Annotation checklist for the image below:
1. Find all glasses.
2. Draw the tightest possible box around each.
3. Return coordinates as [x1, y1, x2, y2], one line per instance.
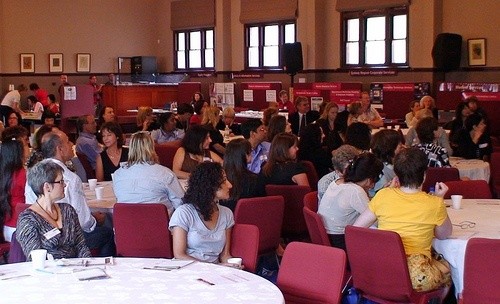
[9, 117, 18, 120]
[43, 180, 64, 187]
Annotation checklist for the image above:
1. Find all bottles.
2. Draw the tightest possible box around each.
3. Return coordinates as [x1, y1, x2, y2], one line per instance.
[346, 288, 359, 304]
[225, 125, 230, 141]
[430, 187, 434, 196]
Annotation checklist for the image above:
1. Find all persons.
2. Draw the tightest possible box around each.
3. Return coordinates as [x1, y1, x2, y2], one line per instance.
[0, 125, 116, 262]
[406, 96, 492, 167]
[137, 92, 272, 214]
[111, 130, 186, 218]
[317, 122, 452, 304]
[168, 160, 244, 268]
[0, 83, 59, 141]
[86, 73, 104, 108]
[95, 104, 128, 181]
[105, 73, 114, 85]
[59, 74, 68, 87]
[261, 90, 384, 188]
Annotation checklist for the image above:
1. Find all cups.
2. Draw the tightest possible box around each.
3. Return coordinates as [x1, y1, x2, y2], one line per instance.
[95, 187, 104, 200]
[395, 125, 399, 129]
[88, 179, 97, 191]
[451, 195, 463, 209]
[30, 249, 47, 269]
[387, 126, 391, 129]
[227, 258, 242, 267]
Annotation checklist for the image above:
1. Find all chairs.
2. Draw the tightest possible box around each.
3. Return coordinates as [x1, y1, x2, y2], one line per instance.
[0, 112, 500, 304]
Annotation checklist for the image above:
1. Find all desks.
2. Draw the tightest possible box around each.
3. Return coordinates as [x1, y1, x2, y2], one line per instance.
[21, 113, 42, 132]
[370, 128, 451, 139]
[0, 258, 285, 304]
[447, 157, 491, 184]
[369, 198, 500, 300]
[235, 110, 289, 119]
[220, 130, 245, 143]
[81, 179, 188, 214]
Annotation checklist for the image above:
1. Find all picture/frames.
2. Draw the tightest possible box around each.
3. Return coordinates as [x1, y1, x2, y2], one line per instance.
[49, 53, 64, 73]
[76, 53, 91, 73]
[467, 38, 487, 66]
[20, 53, 35, 73]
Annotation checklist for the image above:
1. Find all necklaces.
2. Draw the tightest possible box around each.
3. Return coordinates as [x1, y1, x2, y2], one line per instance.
[36, 200, 58, 221]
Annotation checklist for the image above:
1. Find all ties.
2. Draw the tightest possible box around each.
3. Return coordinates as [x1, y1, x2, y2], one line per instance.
[300, 115, 305, 137]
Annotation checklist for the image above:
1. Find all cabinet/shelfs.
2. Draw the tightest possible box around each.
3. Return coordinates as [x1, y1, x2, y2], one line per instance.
[101, 82, 201, 112]
